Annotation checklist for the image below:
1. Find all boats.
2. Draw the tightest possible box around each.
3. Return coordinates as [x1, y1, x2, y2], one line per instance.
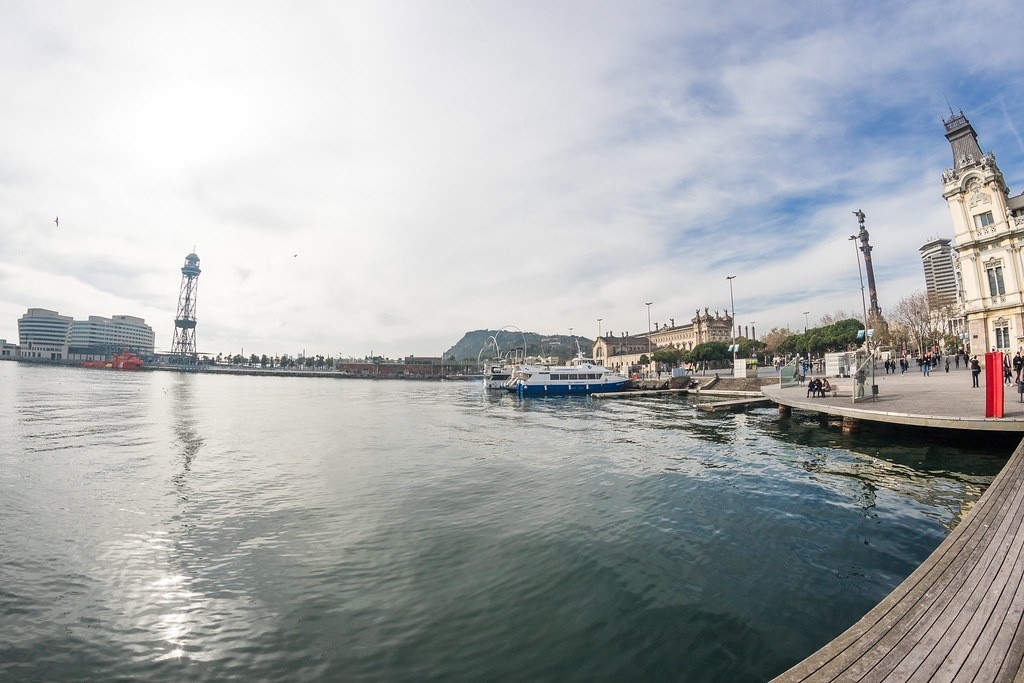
[484, 337, 631, 400]
[79, 347, 143, 369]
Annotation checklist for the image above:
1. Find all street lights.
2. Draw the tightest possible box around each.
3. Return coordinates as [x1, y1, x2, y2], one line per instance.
[803, 312, 810, 330]
[568, 327, 573, 361]
[645, 302, 653, 378]
[848, 235, 872, 358]
[727, 276, 736, 362]
[597, 319, 602, 364]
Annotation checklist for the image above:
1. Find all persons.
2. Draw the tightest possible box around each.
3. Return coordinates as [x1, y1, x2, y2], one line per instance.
[964, 351, 968, 367]
[885, 358, 895, 374]
[775, 360, 781, 371]
[1013, 347, 1024, 383]
[955, 352, 959, 368]
[916, 349, 942, 377]
[1004, 354, 1014, 387]
[799, 361, 813, 387]
[855, 370, 866, 399]
[945, 356, 951, 372]
[807, 378, 831, 398]
[900, 357, 908, 376]
[969, 355, 979, 388]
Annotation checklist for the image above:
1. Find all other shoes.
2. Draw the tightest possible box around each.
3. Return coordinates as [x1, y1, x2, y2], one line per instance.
[854, 397, 858, 399]
[1009, 383, 1014, 386]
[1014, 382, 1018, 386]
[1004, 383, 1008, 387]
[812, 396, 815, 398]
[817, 396, 819, 398]
[977, 386, 979, 387]
[859, 395, 863, 399]
[972, 386, 975, 388]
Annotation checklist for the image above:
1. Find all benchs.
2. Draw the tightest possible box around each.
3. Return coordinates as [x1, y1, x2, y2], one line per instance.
[814, 384, 839, 399]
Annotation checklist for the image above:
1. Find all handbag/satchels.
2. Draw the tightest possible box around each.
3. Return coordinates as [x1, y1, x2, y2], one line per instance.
[1012, 365, 1018, 371]
[1003, 366, 1007, 371]
[977, 365, 981, 374]
